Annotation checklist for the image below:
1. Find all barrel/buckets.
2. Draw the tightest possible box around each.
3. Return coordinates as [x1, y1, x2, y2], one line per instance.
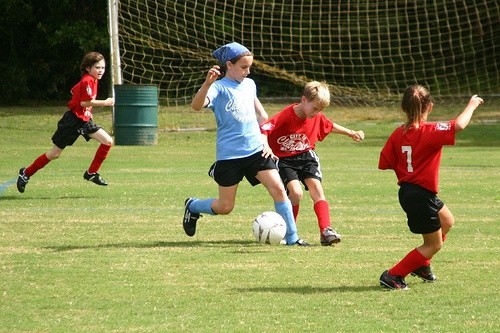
[113, 83, 159, 145]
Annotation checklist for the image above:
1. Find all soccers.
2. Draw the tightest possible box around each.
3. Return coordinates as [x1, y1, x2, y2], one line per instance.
[251, 211, 287, 245]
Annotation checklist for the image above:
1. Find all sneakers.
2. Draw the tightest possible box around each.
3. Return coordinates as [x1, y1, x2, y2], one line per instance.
[379, 271, 412, 291]
[182, 198, 203, 237]
[320, 227, 341, 246]
[287, 239, 311, 247]
[16, 167, 30, 193]
[83, 170, 108, 186]
[411, 263, 437, 283]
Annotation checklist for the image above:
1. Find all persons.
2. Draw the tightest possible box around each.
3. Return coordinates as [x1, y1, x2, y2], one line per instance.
[258, 81, 365, 245]
[17, 52, 115, 193]
[182, 43, 314, 247]
[380, 86, 484, 290]
